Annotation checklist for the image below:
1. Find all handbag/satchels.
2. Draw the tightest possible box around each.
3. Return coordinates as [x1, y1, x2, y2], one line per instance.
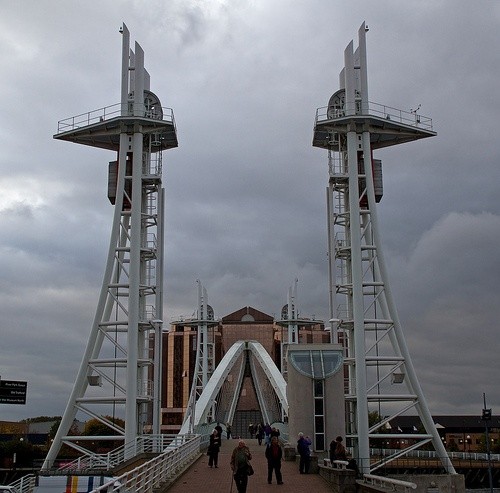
[207, 446, 212, 455]
[246, 460, 254, 476]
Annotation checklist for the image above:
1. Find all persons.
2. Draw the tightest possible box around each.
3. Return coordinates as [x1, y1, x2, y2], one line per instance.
[215, 421, 363, 479]
[208, 429, 220, 468]
[229, 440, 253, 493]
[265, 436, 284, 485]
